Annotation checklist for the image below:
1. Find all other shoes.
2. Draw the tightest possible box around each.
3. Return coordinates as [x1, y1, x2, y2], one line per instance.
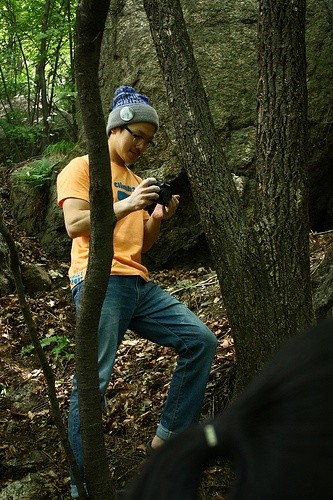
[146, 440, 169, 459]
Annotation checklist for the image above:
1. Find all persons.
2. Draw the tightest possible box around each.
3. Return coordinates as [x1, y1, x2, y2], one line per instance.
[57, 85, 218, 500]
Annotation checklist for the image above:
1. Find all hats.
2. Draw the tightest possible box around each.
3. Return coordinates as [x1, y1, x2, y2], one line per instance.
[106, 85, 159, 137]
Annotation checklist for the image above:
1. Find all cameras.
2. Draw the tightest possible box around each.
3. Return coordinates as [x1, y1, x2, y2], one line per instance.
[148, 178, 174, 206]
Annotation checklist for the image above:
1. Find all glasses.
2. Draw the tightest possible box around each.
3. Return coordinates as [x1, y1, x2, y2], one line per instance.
[124, 126, 155, 148]
